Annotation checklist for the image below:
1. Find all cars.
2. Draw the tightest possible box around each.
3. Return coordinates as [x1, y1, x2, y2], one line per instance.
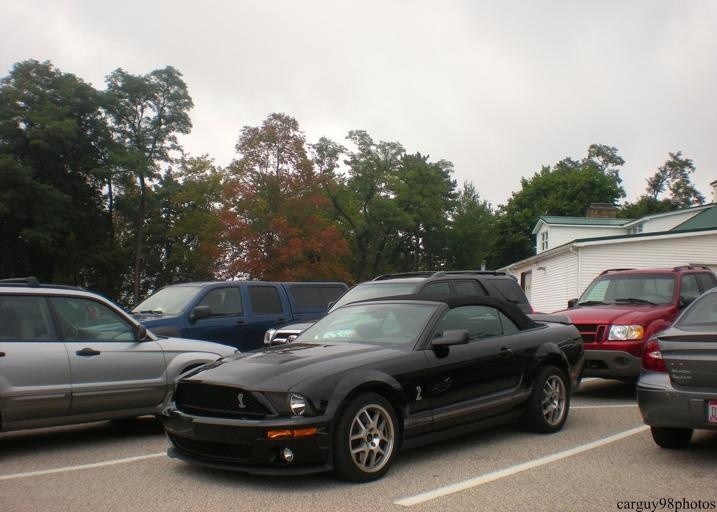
[0, 260, 715, 483]
